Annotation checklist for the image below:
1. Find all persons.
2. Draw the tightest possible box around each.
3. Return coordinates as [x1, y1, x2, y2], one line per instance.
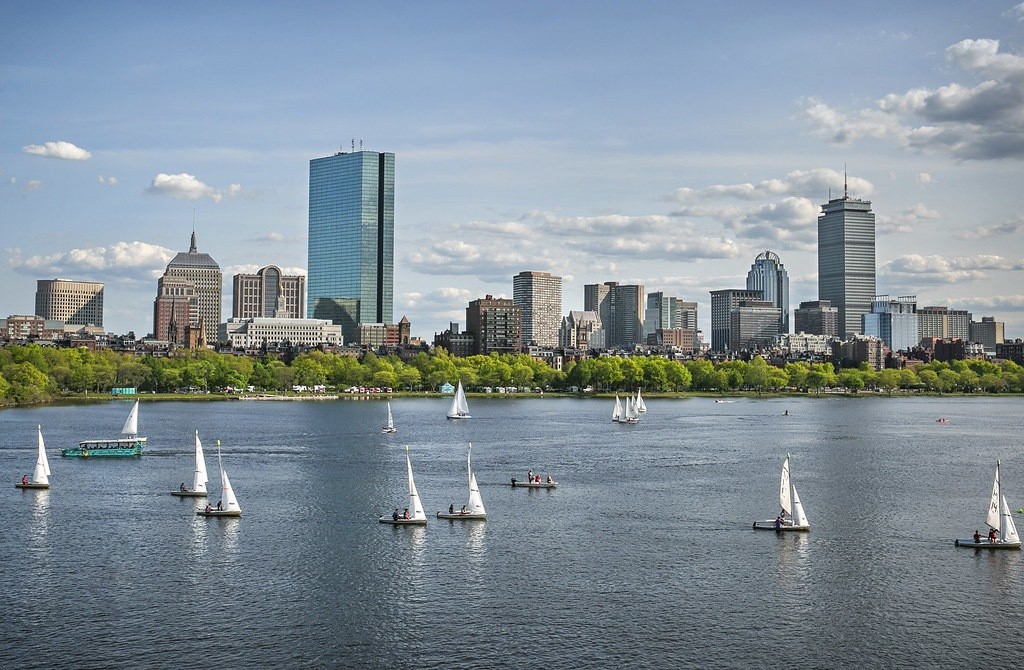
[461, 506, 469, 514]
[774, 517, 783, 526]
[780, 509, 786, 519]
[392, 509, 402, 520]
[988, 528, 998, 542]
[217, 501, 222, 511]
[404, 509, 411, 519]
[22, 475, 28, 484]
[535, 475, 541, 484]
[180, 483, 187, 493]
[205, 502, 213, 513]
[547, 476, 552, 483]
[974, 530, 981, 543]
[528, 470, 534, 482]
[449, 505, 455, 514]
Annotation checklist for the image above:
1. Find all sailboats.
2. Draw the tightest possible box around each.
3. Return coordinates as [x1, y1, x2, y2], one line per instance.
[194, 440, 242, 517]
[435, 444, 487, 519]
[171, 428, 210, 498]
[380, 400, 398, 434]
[15, 425, 54, 488]
[120, 399, 142, 446]
[377, 444, 428, 525]
[446, 379, 473, 420]
[612, 386, 646, 425]
[751, 452, 812, 530]
[954, 458, 1023, 548]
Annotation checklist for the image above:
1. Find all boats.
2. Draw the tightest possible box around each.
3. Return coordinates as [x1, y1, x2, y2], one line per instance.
[59, 436, 149, 460]
[511, 476, 559, 488]
[936, 418, 949, 423]
[713, 399, 727, 404]
